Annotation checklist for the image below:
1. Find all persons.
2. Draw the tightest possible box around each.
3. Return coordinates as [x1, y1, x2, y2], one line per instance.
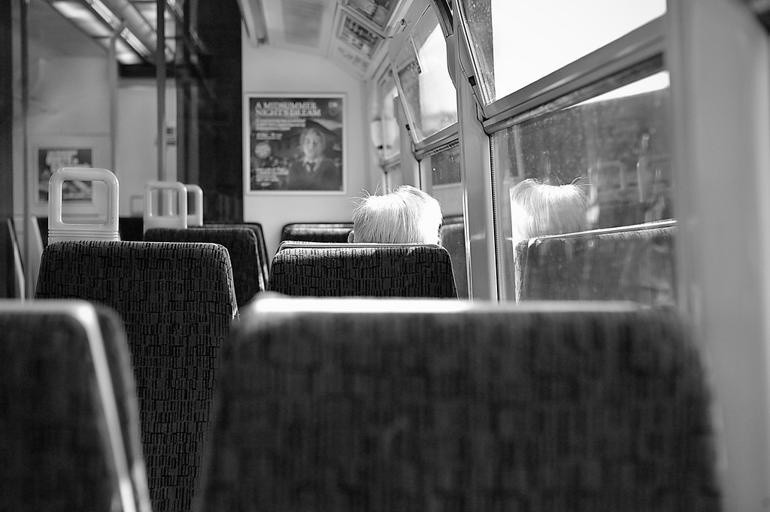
[286, 128, 341, 190]
[347, 186, 444, 245]
[509, 180, 587, 245]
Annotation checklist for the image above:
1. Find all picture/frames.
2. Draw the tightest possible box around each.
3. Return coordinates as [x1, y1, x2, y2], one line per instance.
[32, 142, 94, 206]
[244, 90, 350, 197]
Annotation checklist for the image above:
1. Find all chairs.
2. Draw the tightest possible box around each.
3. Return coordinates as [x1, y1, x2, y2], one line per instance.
[1, 207, 730, 511]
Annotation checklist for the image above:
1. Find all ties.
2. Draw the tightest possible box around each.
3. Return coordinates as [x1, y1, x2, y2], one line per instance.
[307, 161, 316, 173]
